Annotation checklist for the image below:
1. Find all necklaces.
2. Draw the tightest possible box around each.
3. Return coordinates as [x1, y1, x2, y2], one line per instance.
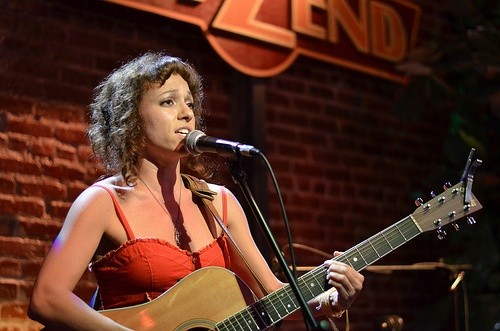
[136, 173, 190, 251]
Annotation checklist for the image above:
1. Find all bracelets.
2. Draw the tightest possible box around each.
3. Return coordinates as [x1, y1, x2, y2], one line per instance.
[315, 286, 347, 320]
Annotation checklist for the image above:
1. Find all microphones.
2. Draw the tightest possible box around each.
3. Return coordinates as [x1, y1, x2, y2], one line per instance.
[185, 130, 260, 158]
[450, 270, 466, 291]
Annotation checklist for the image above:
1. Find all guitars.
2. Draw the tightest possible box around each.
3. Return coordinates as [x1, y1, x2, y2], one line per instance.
[40, 179, 483, 331]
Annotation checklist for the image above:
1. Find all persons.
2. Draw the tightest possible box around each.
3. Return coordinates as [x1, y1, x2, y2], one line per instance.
[26, 51, 365, 331]
